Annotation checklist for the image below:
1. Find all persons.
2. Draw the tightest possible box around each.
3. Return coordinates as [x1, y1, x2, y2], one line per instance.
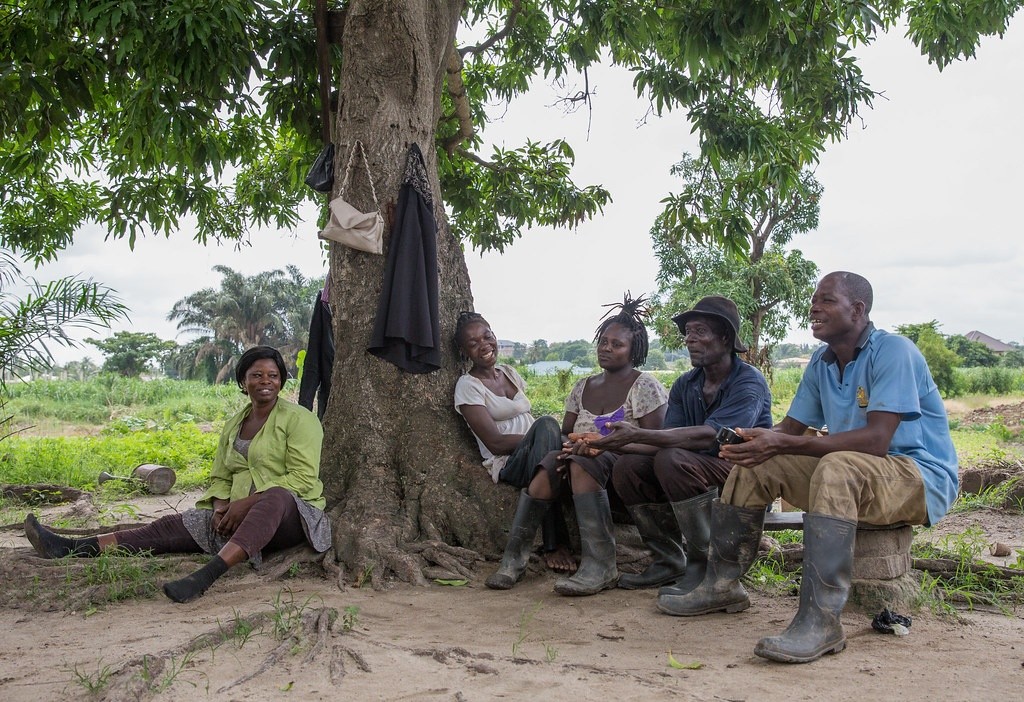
[25, 346, 331, 602]
[656, 270, 958, 661]
[571, 296, 773, 594]
[484, 289, 669, 595]
[453, 311, 577, 570]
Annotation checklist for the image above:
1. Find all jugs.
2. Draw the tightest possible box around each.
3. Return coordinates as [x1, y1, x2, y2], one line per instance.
[98, 463, 176, 494]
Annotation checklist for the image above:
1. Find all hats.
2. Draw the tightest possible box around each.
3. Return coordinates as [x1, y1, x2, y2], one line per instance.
[672, 296, 748, 355]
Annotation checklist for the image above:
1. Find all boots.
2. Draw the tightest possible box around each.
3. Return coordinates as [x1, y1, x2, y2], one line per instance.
[657, 484, 719, 598]
[656, 497, 769, 616]
[753, 512, 859, 663]
[485, 487, 556, 590]
[619, 503, 689, 590]
[555, 489, 619, 595]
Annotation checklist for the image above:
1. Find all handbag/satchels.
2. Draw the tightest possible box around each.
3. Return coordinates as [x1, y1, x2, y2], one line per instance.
[318, 139, 385, 254]
[303, 142, 336, 192]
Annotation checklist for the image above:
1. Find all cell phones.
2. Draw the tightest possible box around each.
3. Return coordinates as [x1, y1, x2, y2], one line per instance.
[716, 425, 746, 444]
